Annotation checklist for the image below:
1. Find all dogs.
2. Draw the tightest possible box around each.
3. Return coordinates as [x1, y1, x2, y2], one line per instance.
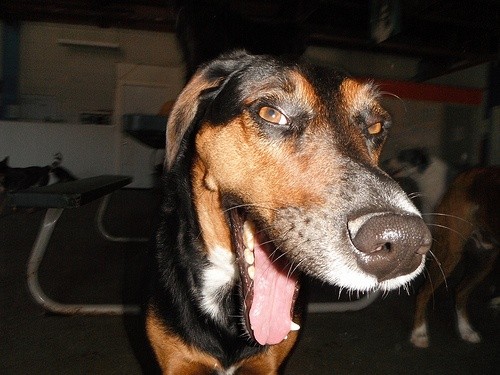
[409, 164, 499, 349]
[0, 154, 77, 214]
[144, 48, 481, 375]
[379, 144, 495, 252]
